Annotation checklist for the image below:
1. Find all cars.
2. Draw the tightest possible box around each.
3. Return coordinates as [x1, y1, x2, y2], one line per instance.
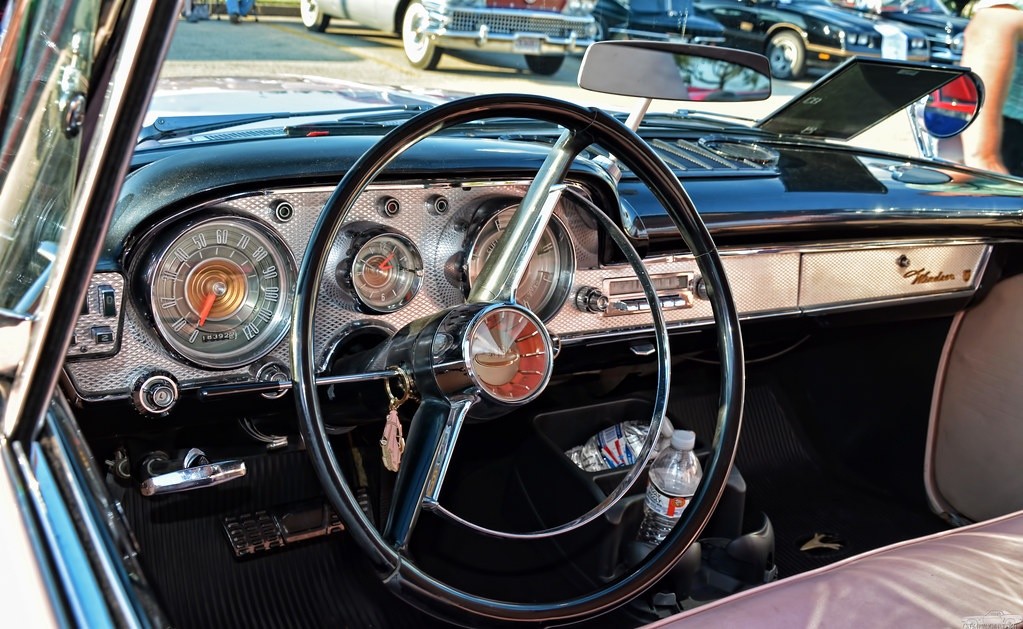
[587, 0, 971, 80]
[301, 0, 601, 76]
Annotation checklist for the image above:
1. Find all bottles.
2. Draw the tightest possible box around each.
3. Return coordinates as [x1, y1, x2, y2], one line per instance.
[636, 430, 702, 544]
[564, 415, 675, 472]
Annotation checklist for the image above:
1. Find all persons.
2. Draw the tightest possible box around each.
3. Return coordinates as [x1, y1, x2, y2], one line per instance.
[958, 0, 1023, 179]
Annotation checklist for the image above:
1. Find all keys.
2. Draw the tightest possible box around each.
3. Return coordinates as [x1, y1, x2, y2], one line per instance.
[379, 365, 411, 472]
